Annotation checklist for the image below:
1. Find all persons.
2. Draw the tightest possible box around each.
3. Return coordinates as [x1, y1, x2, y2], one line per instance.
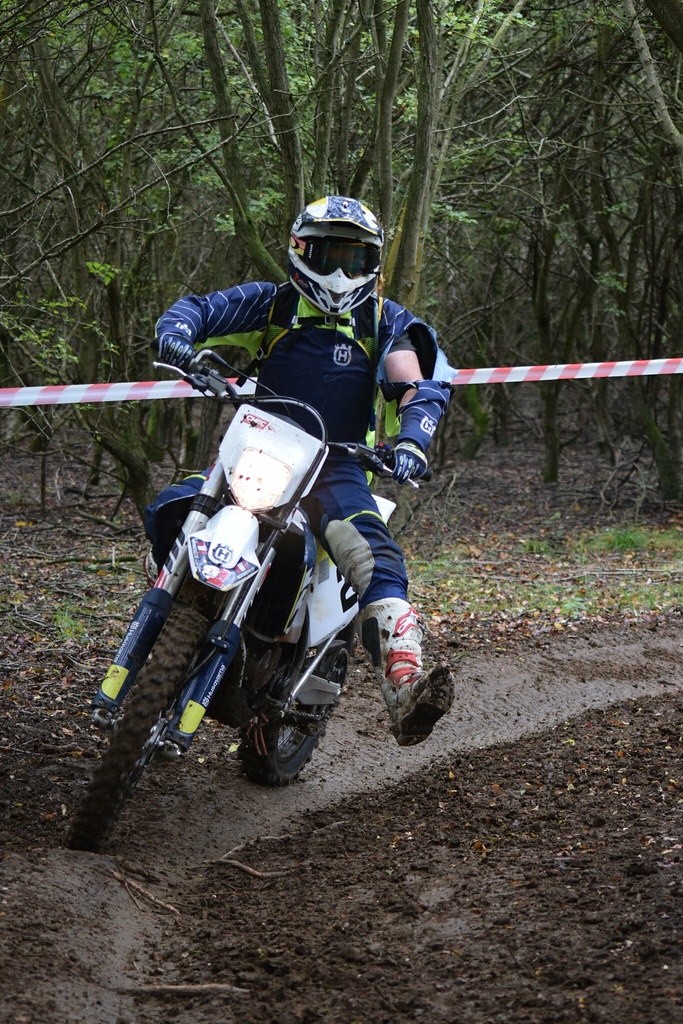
[144, 196, 455, 746]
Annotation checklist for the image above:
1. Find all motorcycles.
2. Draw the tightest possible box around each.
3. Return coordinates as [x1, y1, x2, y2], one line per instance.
[64, 338, 433, 855]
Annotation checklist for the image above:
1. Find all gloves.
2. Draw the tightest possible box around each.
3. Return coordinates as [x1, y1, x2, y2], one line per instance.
[156, 333, 195, 366]
[390, 440, 430, 484]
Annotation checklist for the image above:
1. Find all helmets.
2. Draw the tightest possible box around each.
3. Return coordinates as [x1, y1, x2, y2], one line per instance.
[287, 195, 385, 316]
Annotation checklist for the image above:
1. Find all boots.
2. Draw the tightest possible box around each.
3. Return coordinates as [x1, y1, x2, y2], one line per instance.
[363, 596, 453, 747]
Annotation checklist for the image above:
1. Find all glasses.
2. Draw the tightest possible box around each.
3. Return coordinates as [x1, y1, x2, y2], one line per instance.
[297, 238, 378, 280]
